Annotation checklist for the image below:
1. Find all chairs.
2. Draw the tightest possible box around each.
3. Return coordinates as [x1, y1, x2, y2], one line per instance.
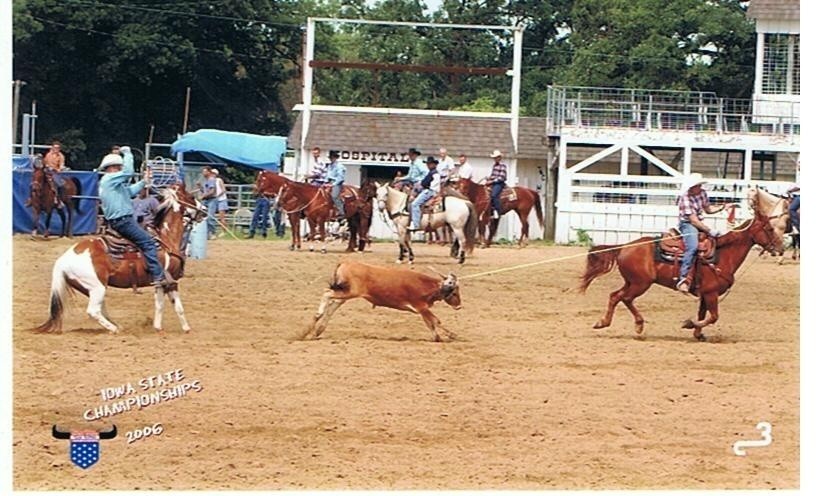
[231, 209, 252, 239]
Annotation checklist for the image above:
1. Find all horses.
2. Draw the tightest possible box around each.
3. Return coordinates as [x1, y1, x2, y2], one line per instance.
[31, 162, 83, 240]
[28, 183, 208, 338]
[251, 168, 544, 270]
[746, 183, 799, 264]
[580, 207, 786, 343]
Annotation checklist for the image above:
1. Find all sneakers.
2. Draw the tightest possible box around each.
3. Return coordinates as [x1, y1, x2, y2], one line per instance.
[155, 278, 180, 286]
[244, 233, 255, 240]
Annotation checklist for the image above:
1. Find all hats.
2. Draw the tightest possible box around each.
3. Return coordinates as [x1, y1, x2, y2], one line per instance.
[406, 148, 421, 156]
[210, 168, 220, 177]
[423, 156, 440, 165]
[98, 153, 127, 171]
[489, 149, 505, 159]
[680, 171, 707, 191]
[327, 150, 340, 159]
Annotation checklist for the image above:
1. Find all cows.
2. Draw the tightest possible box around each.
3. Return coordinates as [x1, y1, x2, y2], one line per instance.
[301, 259, 463, 344]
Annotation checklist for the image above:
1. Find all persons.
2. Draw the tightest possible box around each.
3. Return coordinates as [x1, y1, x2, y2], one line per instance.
[96, 144, 289, 240]
[675, 172, 733, 293]
[91, 146, 173, 286]
[407, 156, 442, 232]
[300, 147, 328, 186]
[448, 153, 474, 181]
[485, 149, 508, 220]
[783, 160, 800, 236]
[304, 149, 346, 220]
[393, 147, 431, 186]
[435, 147, 456, 188]
[23, 141, 66, 208]
